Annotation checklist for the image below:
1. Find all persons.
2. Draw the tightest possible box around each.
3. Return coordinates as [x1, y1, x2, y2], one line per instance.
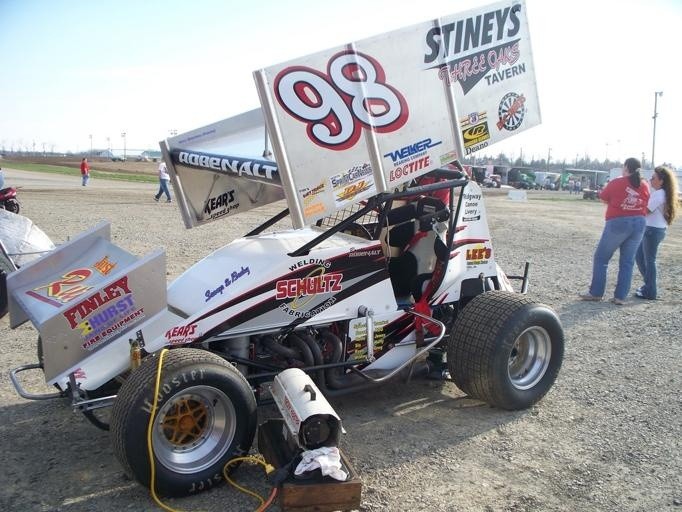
[79, 157, 90, 186]
[403, 164, 453, 207]
[153, 157, 173, 203]
[634, 165, 677, 300]
[0, 166, 4, 191]
[544, 174, 581, 195]
[578, 157, 651, 304]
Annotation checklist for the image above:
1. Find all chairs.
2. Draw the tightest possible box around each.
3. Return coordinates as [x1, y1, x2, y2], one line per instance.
[378, 197, 447, 247]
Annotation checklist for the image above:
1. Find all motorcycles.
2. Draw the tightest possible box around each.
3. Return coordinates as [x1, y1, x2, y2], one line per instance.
[1, 187, 20, 216]
[6, 2, 565, 496]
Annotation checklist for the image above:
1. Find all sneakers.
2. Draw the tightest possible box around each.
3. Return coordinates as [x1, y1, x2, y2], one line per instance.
[609, 296, 624, 304]
[636, 287, 644, 297]
[580, 294, 602, 301]
